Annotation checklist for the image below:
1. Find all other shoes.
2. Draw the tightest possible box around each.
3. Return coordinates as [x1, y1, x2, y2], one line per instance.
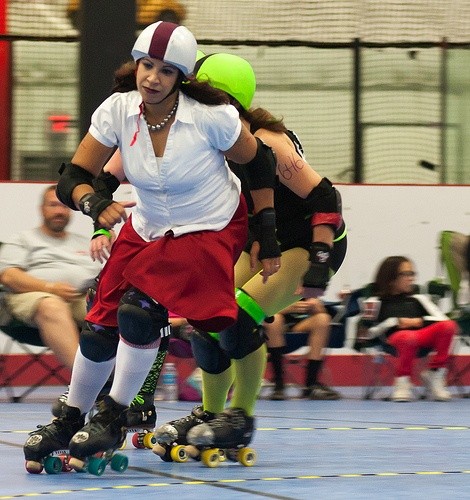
[420, 366, 454, 400]
[268, 380, 293, 400]
[388, 375, 418, 402]
[301, 383, 340, 401]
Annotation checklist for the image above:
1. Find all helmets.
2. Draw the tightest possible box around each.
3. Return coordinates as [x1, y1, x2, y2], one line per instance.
[182, 52, 256, 111]
[131, 21, 197, 80]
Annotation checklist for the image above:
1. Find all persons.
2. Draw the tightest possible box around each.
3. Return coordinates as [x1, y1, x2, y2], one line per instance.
[91, 62, 166, 449]
[24, 22, 281, 476]
[152, 52, 348, 467]
[154, 311, 202, 400]
[0, 185, 110, 372]
[358, 257, 458, 401]
[263, 300, 340, 401]
[51, 49, 207, 448]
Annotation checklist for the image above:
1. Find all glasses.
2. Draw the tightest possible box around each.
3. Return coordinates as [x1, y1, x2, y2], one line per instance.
[395, 271, 416, 277]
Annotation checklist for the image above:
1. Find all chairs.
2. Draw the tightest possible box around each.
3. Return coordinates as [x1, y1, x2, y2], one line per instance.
[0, 278, 470, 403]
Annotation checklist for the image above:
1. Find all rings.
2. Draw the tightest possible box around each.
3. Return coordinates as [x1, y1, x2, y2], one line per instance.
[276, 264, 280, 266]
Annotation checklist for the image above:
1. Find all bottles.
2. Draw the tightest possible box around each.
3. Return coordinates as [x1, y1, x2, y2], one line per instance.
[160, 362, 179, 400]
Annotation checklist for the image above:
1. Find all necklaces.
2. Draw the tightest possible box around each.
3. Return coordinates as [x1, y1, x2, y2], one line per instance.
[143, 97, 178, 130]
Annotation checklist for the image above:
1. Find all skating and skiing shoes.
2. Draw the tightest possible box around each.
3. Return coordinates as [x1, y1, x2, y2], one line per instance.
[62, 396, 134, 477]
[151, 407, 222, 464]
[170, 406, 258, 468]
[51, 383, 74, 420]
[118, 393, 159, 449]
[21, 402, 87, 475]
